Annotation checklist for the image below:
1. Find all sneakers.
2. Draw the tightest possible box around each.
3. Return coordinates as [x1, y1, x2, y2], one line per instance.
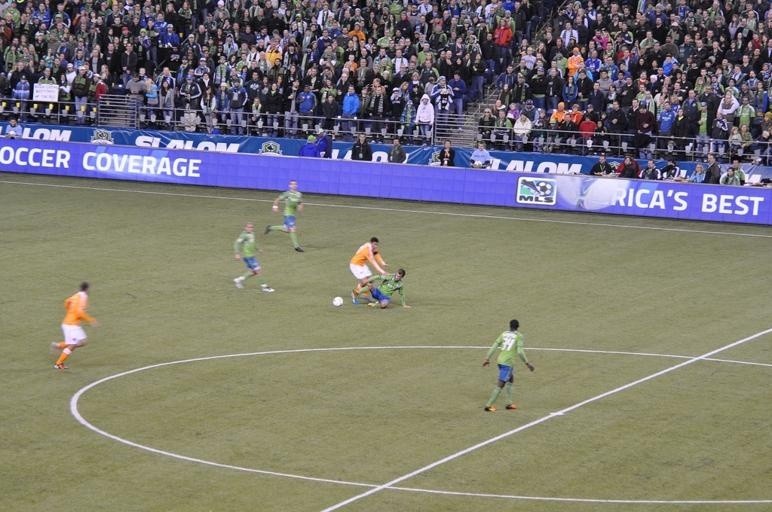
[264, 225, 271, 235]
[50, 341, 59, 353]
[233, 278, 245, 289]
[295, 247, 304, 252]
[505, 403, 519, 411]
[261, 283, 275, 293]
[54, 363, 69, 371]
[351, 288, 380, 307]
[485, 404, 498, 412]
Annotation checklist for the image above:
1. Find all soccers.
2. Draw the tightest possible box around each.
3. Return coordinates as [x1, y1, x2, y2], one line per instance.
[332, 296, 345, 307]
[536, 182, 553, 197]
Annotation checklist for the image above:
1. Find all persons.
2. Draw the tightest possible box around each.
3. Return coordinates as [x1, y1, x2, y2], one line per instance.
[481, 318, 536, 412]
[701, 154, 721, 185]
[719, 167, 742, 186]
[591, 153, 612, 176]
[637, 159, 661, 179]
[351, 268, 411, 309]
[0, 0, 771, 161]
[612, 153, 640, 174]
[439, 140, 455, 167]
[349, 235, 389, 305]
[469, 142, 491, 170]
[688, 165, 706, 184]
[733, 159, 747, 186]
[658, 160, 679, 180]
[619, 154, 639, 177]
[49, 283, 99, 374]
[264, 179, 305, 254]
[232, 221, 275, 293]
